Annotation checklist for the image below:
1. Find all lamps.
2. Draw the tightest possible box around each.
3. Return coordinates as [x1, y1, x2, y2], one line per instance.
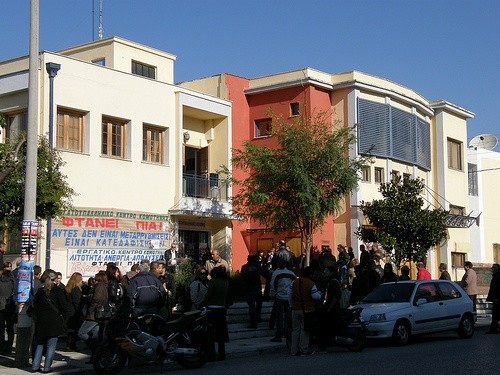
[183, 131, 189, 140]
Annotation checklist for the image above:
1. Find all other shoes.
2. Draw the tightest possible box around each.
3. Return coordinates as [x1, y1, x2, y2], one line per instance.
[485, 330, 497, 334]
[271, 337, 282, 342]
[32, 369, 41, 372]
[44, 370, 53, 372]
[248, 324, 256, 328]
[301, 351, 314, 356]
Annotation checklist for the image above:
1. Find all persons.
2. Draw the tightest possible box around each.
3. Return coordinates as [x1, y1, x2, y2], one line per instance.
[65, 272, 84, 351]
[201, 266, 230, 363]
[438, 263, 452, 281]
[322, 278, 343, 314]
[26, 273, 68, 373]
[241, 253, 269, 329]
[416, 262, 431, 281]
[77, 273, 108, 365]
[457, 262, 477, 324]
[381, 263, 398, 283]
[0, 241, 7, 269]
[0, 262, 17, 356]
[398, 266, 412, 281]
[80, 242, 231, 329]
[269, 257, 298, 343]
[289, 266, 321, 357]
[255, 240, 381, 300]
[28, 266, 67, 357]
[485, 264, 500, 334]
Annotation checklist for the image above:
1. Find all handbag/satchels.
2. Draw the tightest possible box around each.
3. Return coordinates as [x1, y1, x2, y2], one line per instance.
[55, 313, 67, 334]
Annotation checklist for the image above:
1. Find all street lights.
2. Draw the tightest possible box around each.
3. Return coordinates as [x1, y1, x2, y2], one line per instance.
[45, 62, 62, 268]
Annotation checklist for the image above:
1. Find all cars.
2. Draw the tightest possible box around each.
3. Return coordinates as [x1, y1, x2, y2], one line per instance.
[345, 279, 478, 346]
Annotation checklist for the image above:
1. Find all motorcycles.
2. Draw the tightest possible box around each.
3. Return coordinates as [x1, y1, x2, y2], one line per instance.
[91, 301, 214, 375]
[285, 307, 369, 353]
